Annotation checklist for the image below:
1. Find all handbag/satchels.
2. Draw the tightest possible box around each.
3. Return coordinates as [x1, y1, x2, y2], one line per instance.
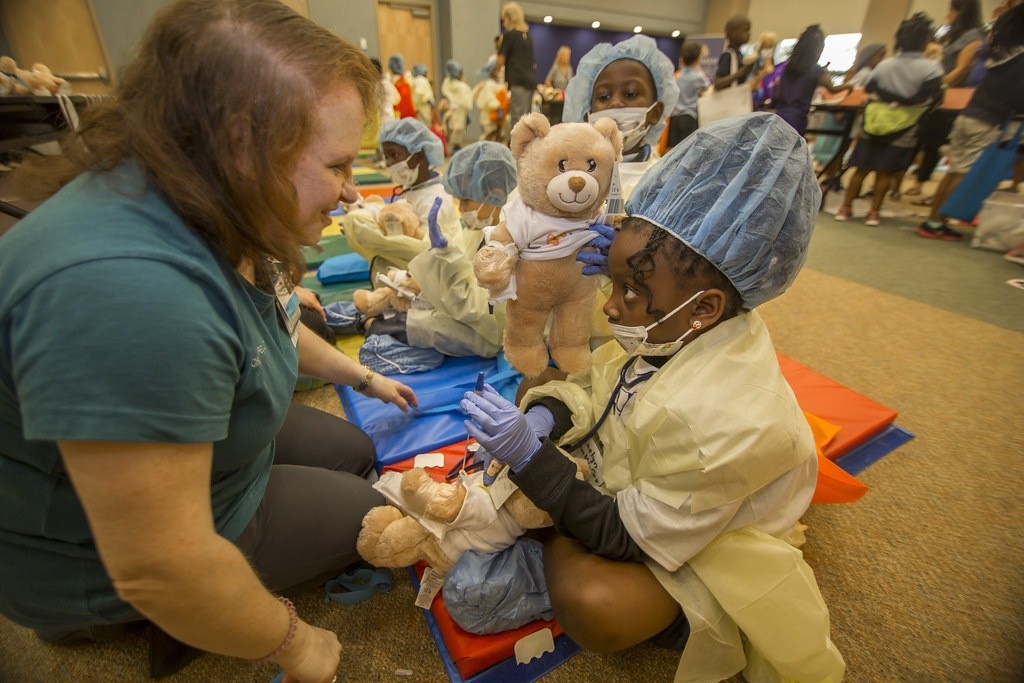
[863, 98, 935, 144]
[698, 45, 753, 127]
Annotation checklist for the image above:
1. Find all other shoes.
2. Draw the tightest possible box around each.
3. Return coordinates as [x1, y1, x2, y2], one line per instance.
[834, 208, 852, 221]
[865, 210, 881, 226]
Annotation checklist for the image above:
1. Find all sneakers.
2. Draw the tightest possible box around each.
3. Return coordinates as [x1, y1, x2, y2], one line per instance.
[916, 221, 964, 241]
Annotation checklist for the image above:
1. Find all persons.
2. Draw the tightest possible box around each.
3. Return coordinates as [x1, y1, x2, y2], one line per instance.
[716, 1, 1024, 241]
[344, 35, 846, 683]
[0, 1, 419, 683]
[491, 3, 536, 128]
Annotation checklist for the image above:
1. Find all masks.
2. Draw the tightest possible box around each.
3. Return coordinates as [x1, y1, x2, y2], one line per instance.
[606, 290, 712, 357]
[460, 193, 497, 231]
[383, 150, 422, 190]
[588, 102, 660, 152]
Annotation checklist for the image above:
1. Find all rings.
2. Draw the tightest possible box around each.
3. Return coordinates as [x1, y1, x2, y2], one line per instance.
[332, 675, 337, 683]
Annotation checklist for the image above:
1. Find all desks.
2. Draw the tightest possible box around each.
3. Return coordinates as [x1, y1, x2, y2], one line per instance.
[758, 87, 975, 213]
[0, 91, 114, 220]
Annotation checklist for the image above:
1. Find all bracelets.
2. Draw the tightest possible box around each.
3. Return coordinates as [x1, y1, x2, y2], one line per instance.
[254, 596, 298, 664]
[352, 370, 373, 393]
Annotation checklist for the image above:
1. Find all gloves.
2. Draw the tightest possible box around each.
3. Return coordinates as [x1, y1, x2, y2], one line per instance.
[460, 382, 543, 472]
[474, 407, 555, 486]
[497, 107, 502, 118]
[427, 196, 448, 248]
[466, 114, 472, 125]
[429, 100, 434, 108]
[576, 224, 616, 276]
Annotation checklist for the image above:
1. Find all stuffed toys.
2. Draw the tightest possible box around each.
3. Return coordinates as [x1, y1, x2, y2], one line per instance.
[358, 447, 590, 575]
[339, 194, 423, 241]
[0, 56, 61, 87]
[476, 113, 623, 376]
[354, 265, 421, 317]
